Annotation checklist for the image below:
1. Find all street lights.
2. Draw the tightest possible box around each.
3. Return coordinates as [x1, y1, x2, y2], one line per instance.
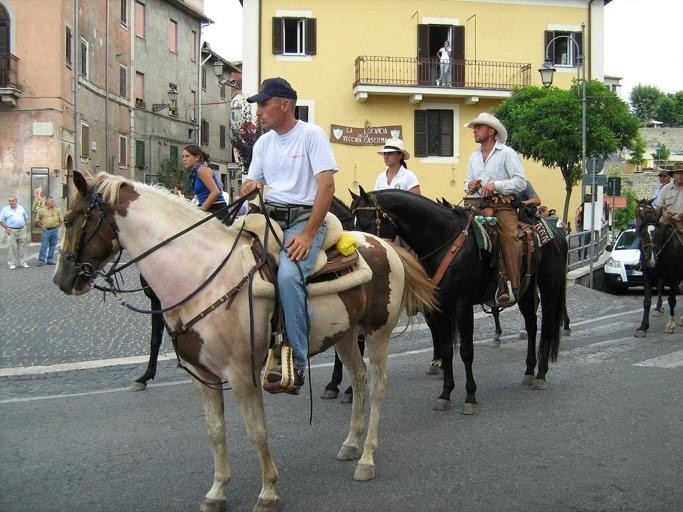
[537, 32, 588, 261]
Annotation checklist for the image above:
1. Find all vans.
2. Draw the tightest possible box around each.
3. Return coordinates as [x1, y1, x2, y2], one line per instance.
[601, 225, 656, 296]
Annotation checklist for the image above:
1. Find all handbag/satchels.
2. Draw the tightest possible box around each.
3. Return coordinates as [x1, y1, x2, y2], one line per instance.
[263, 202, 313, 229]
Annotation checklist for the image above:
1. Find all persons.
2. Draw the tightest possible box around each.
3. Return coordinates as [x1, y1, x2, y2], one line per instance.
[33, 196, 63, 265]
[217, 181, 228, 205]
[0, 196, 29, 269]
[632, 161, 682, 273]
[461, 112, 527, 306]
[171, 184, 181, 195]
[228, 195, 244, 217]
[575, 203, 589, 262]
[435, 41, 452, 87]
[182, 145, 228, 223]
[372, 139, 419, 194]
[652, 170, 671, 208]
[547, 208, 556, 218]
[237, 77, 338, 394]
[539, 206, 545, 218]
[31, 185, 47, 227]
[515, 179, 541, 208]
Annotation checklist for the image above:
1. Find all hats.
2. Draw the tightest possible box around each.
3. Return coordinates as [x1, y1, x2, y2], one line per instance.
[668, 163, 683, 178]
[657, 171, 668, 176]
[464, 112, 508, 144]
[247, 77, 297, 103]
[376, 138, 410, 160]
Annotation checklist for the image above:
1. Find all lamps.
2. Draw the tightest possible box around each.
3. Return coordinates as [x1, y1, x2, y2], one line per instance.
[153, 87, 178, 112]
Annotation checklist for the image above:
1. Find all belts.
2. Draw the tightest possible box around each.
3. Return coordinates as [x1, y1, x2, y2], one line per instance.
[10, 227, 23, 229]
[46, 227, 57, 230]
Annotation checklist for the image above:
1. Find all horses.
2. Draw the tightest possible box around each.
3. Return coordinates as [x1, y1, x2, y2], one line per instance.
[348, 186, 568, 415]
[634, 209, 683, 338]
[53, 171, 444, 512]
[631, 196, 659, 235]
[319, 180, 444, 404]
[435, 196, 571, 348]
[125, 272, 164, 393]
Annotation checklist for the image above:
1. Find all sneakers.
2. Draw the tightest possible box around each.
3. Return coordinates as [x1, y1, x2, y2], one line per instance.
[499, 288, 519, 302]
[268, 366, 304, 395]
[9, 261, 56, 270]
